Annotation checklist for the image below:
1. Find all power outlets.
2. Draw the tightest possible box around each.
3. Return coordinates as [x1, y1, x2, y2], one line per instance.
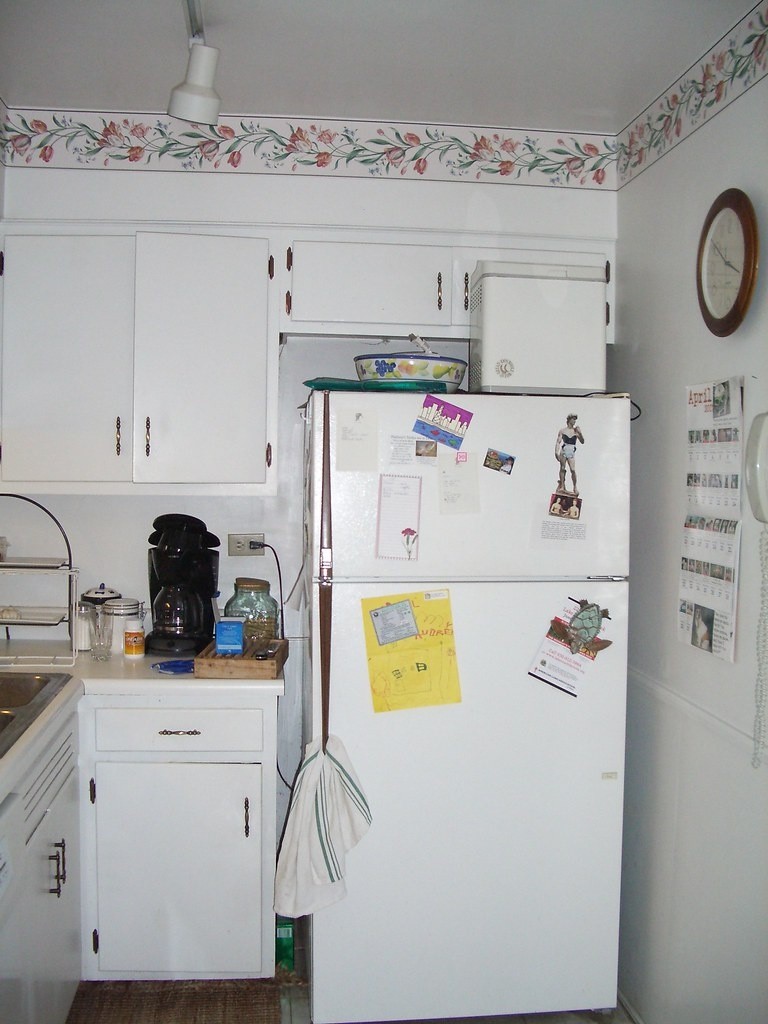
[228, 533, 265, 557]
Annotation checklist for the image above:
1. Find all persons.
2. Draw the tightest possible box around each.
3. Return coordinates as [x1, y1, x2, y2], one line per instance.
[551, 498, 579, 518]
[500, 456, 515, 475]
[554, 413, 585, 495]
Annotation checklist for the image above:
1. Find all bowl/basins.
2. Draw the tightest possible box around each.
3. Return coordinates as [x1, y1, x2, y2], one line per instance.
[354, 352, 468, 394]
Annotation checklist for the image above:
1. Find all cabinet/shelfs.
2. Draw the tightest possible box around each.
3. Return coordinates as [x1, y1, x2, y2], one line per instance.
[281, 225, 617, 346]
[0, 221, 276, 498]
[0, 694, 278, 1024]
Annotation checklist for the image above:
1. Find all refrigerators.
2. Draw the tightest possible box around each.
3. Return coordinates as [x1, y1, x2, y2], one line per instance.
[300, 389, 631, 1024]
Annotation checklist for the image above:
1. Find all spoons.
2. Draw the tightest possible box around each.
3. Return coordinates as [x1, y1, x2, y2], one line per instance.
[244, 634, 280, 660]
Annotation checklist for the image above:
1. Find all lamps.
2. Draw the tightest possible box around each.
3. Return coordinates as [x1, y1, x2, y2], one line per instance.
[166, 0, 222, 127]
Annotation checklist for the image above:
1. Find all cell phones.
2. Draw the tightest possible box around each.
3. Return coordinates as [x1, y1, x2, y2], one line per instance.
[256, 649, 267, 660]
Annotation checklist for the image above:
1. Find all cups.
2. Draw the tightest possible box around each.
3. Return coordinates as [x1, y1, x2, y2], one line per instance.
[89, 609, 113, 662]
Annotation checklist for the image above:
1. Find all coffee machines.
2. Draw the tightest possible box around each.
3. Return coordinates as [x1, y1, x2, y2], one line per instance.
[143, 513, 220, 656]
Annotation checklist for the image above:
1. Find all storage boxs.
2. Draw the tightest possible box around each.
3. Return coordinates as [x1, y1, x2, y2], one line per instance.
[470, 259, 607, 399]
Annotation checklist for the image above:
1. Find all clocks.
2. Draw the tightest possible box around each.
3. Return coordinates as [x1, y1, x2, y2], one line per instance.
[695, 188, 759, 338]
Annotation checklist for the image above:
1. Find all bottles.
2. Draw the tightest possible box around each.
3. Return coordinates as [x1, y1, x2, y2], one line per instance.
[81, 583, 146, 660]
[71, 601, 96, 650]
[224, 577, 278, 639]
